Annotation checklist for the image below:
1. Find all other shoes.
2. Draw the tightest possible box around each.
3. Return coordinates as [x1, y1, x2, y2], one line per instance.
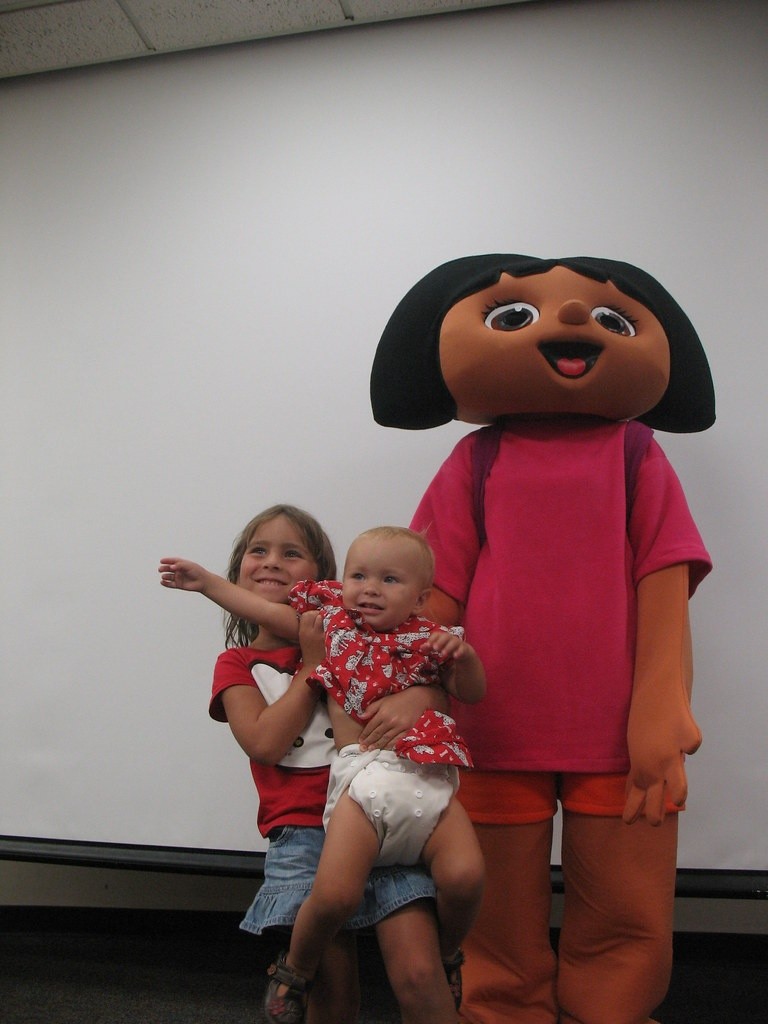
[265, 951, 319, 1024]
[441, 947, 464, 1011]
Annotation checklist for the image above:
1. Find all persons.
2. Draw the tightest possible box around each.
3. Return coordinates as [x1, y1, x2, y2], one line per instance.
[158, 525, 484, 1024]
[208, 504, 458, 1024]
[369, 253, 716, 1024]
